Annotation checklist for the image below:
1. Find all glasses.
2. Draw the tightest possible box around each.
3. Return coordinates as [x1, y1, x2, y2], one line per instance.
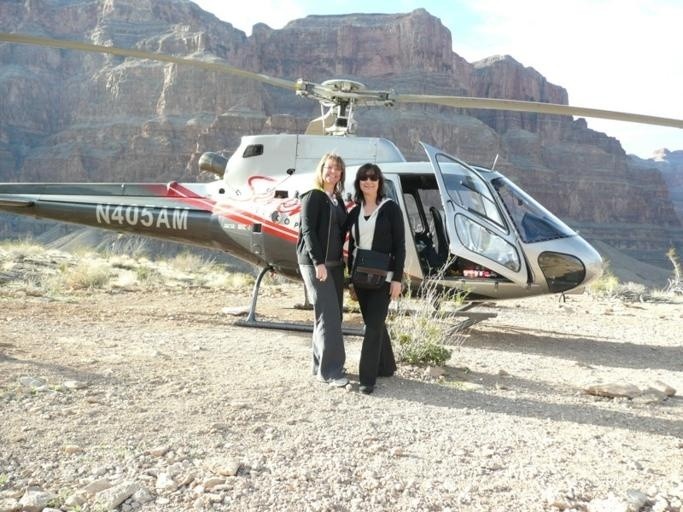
[360, 174, 379, 181]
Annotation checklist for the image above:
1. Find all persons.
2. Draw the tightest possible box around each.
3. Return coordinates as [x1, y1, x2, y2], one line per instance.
[293, 152, 351, 387]
[346, 163, 407, 394]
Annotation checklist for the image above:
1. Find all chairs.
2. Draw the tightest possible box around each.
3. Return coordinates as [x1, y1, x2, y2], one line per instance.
[430, 207, 476, 276]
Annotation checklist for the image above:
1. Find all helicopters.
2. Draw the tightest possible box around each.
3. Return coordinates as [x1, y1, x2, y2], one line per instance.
[0, 33, 683, 341]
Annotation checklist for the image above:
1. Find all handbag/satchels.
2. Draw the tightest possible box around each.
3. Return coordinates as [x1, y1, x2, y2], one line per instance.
[352, 249, 390, 290]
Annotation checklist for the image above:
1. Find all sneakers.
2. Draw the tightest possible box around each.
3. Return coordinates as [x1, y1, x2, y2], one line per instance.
[377, 371, 394, 377]
[316, 373, 348, 386]
[313, 363, 346, 376]
[360, 384, 374, 394]
